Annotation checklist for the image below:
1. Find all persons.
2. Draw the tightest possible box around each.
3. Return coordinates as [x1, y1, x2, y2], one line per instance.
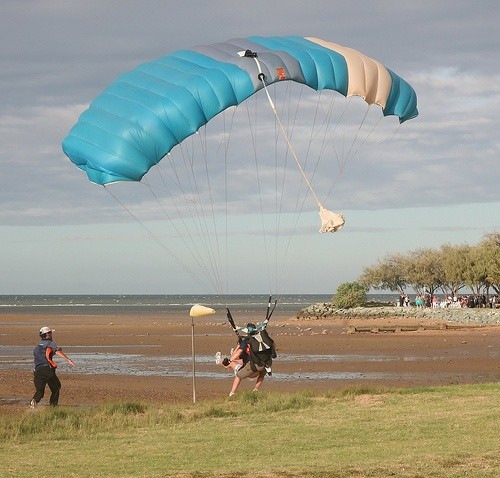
[228, 323, 276, 396]
[30, 326, 77, 410]
[215, 343, 260, 379]
[398, 291, 500, 310]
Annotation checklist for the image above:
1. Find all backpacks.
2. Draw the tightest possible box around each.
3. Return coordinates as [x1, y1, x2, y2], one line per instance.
[251, 331, 273, 363]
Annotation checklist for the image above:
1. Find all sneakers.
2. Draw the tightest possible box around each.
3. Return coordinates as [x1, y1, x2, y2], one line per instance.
[29, 399, 37, 412]
[230, 348, 235, 355]
[252, 388, 259, 391]
[215, 352, 221, 364]
[228, 392, 234, 397]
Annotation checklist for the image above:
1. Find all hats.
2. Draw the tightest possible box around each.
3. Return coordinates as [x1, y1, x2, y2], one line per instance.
[39, 326, 55, 335]
